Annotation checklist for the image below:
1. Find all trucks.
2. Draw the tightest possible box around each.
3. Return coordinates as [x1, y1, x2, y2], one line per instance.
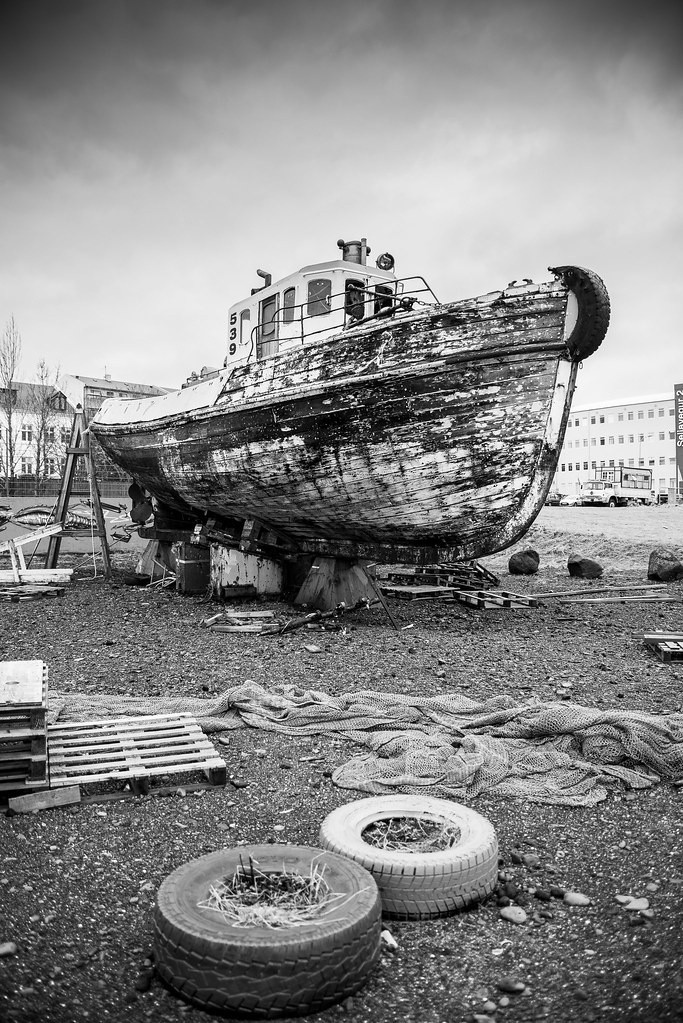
[578, 466, 653, 508]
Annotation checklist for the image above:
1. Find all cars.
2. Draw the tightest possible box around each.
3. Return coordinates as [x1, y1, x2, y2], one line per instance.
[655, 494, 668, 505]
[560, 494, 582, 507]
[546, 493, 559, 506]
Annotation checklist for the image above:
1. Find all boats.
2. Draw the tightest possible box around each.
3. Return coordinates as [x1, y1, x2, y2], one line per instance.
[87, 235, 611, 563]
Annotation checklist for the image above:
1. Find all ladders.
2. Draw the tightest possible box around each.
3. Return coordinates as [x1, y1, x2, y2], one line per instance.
[0, 521, 75, 584]
[45, 406, 114, 581]
